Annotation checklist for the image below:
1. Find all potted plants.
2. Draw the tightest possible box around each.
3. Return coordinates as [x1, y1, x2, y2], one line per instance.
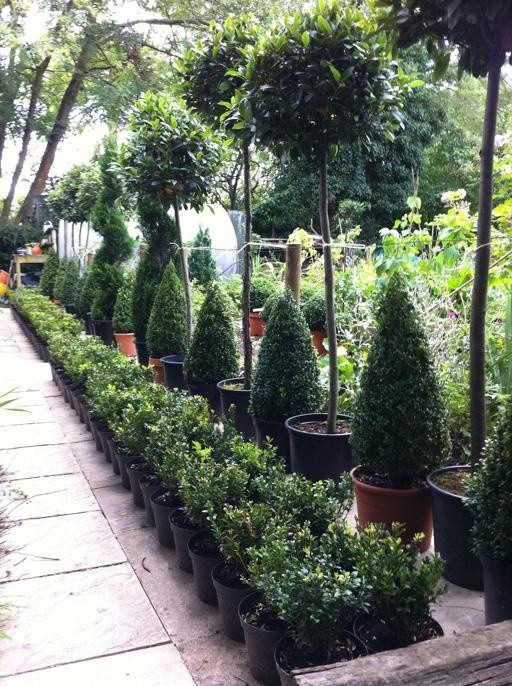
[377, 0, 511, 603]
[52, 93, 234, 401]
[223, 2, 408, 500]
[10, 282, 86, 368]
[345, 260, 457, 554]
[170, 6, 282, 447]
[463, 393, 512, 628]
[50, 326, 445, 686]
